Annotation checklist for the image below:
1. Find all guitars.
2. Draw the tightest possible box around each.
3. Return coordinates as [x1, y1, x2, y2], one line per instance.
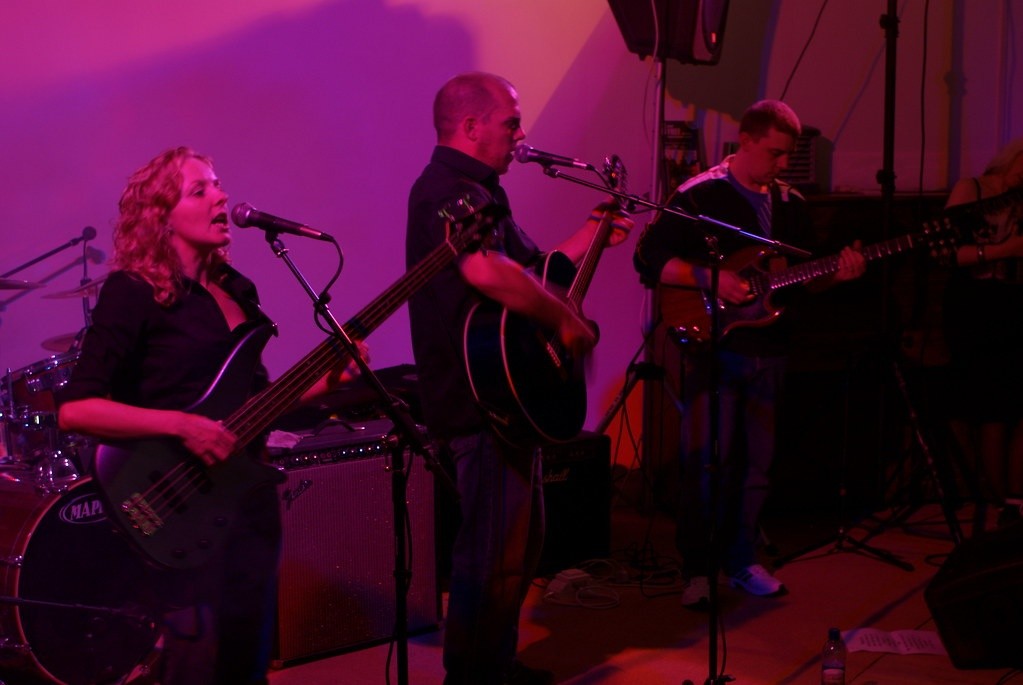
[91, 191, 504, 578]
[461, 152, 629, 451]
[658, 215, 964, 350]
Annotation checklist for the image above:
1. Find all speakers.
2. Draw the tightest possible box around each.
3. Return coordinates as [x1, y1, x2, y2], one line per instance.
[923, 521, 1023, 669]
[607, 0, 667, 57]
[262, 418, 442, 671]
[531, 430, 610, 578]
[667, 0, 731, 66]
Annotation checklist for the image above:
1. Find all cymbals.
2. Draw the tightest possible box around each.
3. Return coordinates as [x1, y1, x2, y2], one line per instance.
[41, 330, 86, 352]
[40, 286, 103, 299]
[0, 277, 44, 289]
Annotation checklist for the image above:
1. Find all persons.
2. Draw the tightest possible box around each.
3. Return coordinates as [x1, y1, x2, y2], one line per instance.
[59, 146, 369, 685]
[630, 99, 871, 610]
[404, 69, 634, 685]
[946, 137, 1023, 506]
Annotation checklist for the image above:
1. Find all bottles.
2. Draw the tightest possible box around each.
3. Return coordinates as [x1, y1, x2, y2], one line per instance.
[820, 626, 847, 685]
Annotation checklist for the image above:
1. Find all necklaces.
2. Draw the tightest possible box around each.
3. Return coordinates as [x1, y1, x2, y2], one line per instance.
[977, 243, 984, 260]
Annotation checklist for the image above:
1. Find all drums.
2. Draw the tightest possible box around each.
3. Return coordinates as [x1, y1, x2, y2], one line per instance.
[2, 349, 98, 455]
[1, 467, 170, 685]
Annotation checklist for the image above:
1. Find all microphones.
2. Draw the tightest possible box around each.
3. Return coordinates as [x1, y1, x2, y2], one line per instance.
[513, 143, 594, 171]
[231, 201, 332, 242]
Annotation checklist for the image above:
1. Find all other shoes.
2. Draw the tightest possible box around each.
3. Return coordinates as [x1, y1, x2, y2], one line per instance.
[507, 661, 552, 685]
[735, 565, 786, 597]
[681, 577, 711, 610]
